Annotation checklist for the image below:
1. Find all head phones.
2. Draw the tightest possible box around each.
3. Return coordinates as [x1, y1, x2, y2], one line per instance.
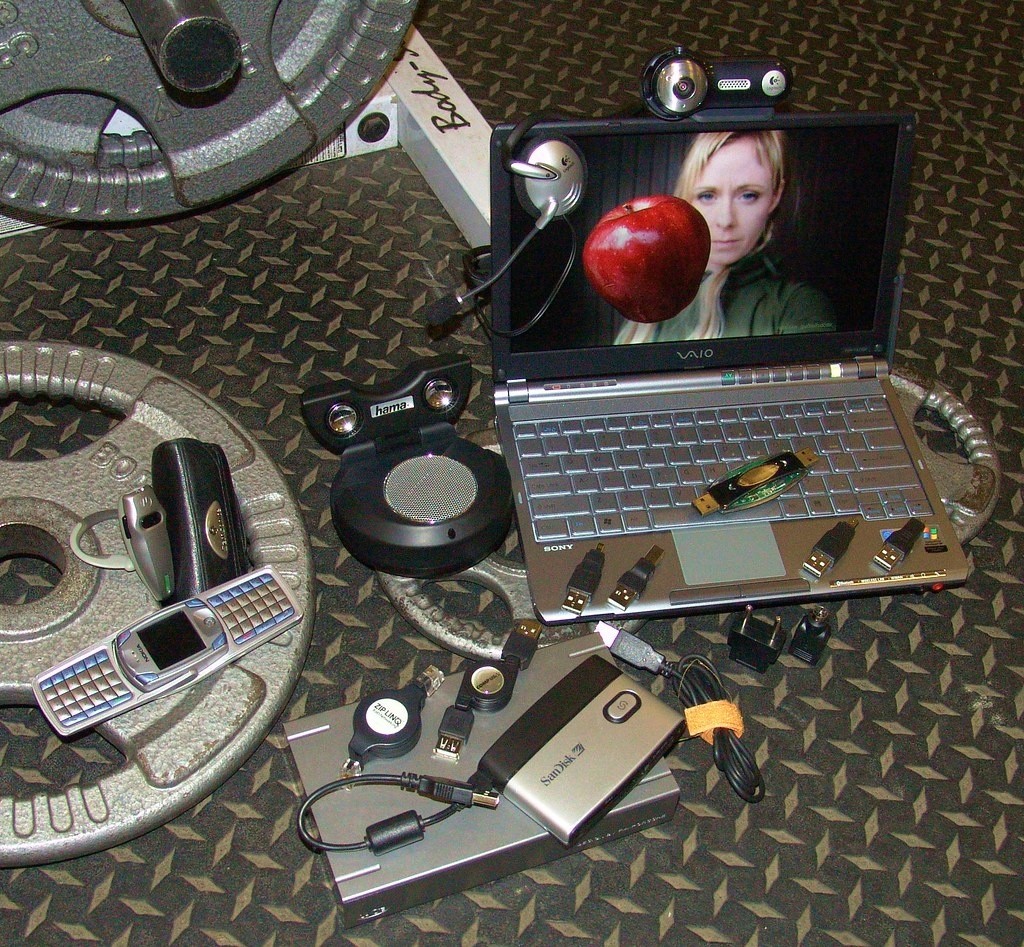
[500, 111, 589, 229]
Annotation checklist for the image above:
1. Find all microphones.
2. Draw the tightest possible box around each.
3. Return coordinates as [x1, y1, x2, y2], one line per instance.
[426, 227, 542, 327]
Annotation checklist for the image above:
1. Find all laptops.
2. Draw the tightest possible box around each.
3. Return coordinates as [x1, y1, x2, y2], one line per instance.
[487, 103, 968, 628]
